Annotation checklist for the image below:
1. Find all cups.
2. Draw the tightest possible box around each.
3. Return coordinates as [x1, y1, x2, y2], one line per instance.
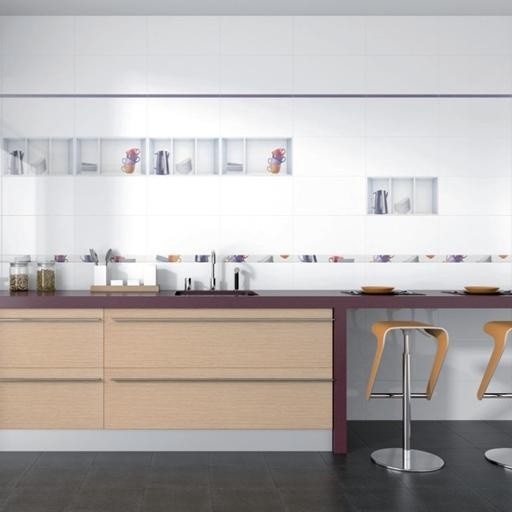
[266, 148, 287, 174]
[371, 189, 389, 214]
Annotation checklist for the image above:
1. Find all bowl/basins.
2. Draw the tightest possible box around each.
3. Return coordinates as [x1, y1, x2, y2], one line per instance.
[394, 198, 410, 214]
[176, 159, 192, 174]
[31, 159, 46, 174]
[120, 148, 141, 173]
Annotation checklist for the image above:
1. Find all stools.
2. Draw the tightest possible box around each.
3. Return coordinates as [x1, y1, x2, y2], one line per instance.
[365, 318, 512, 473]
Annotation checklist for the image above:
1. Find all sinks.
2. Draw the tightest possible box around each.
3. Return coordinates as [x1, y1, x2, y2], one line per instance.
[174, 290, 258, 295]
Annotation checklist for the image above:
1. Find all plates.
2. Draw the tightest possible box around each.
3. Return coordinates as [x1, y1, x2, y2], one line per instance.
[464, 285, 499, 292]
[81, 163, 96, 175]
[227, 161, 244, 174]
[361, 286, 394, 292]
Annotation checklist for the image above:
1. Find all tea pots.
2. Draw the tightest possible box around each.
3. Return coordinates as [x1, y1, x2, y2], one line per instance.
[153, 149, 171, 174]
[7, 150, 25, 175]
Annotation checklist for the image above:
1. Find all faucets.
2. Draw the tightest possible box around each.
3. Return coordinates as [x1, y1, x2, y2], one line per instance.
[209, 251, 216, 293]
